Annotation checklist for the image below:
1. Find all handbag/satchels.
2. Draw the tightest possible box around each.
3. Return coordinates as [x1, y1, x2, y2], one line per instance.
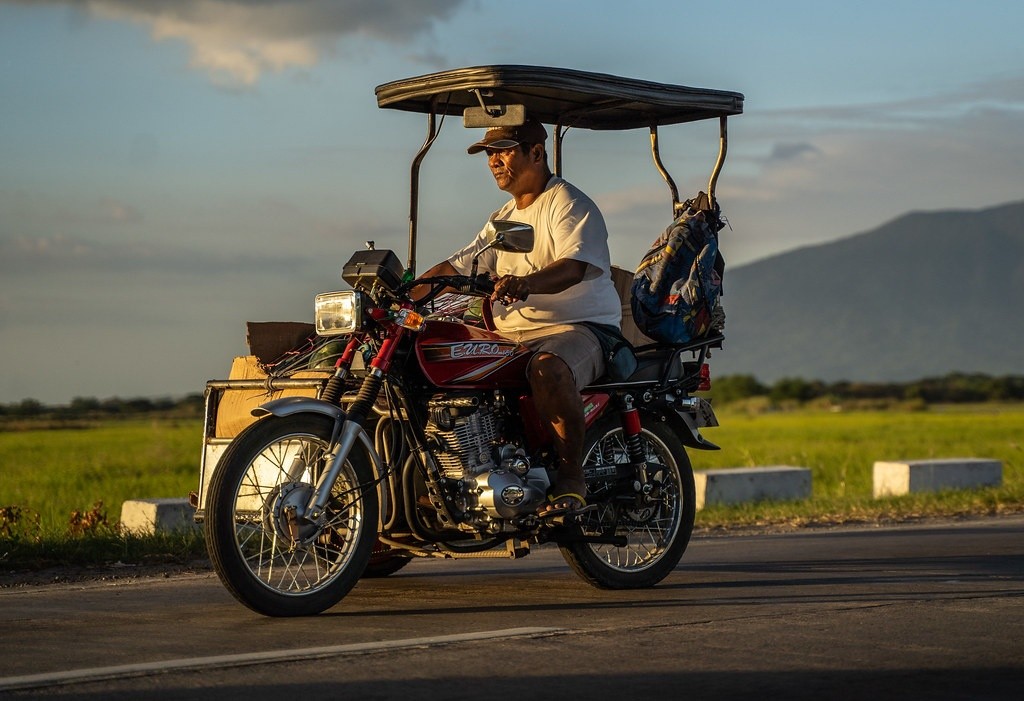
[629, 200, 725, 347]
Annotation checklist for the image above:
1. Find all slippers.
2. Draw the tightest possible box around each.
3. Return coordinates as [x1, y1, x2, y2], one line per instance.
[538, 493, 599, 517]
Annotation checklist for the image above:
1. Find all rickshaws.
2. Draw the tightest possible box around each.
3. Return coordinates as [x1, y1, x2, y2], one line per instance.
[189, 61, 746, 619]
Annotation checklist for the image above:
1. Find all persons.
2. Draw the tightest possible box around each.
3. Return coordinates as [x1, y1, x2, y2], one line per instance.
[407, 117, 627, 516]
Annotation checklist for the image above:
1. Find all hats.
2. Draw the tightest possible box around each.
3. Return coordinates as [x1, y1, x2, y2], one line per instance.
[467, 119, 548, 155]
